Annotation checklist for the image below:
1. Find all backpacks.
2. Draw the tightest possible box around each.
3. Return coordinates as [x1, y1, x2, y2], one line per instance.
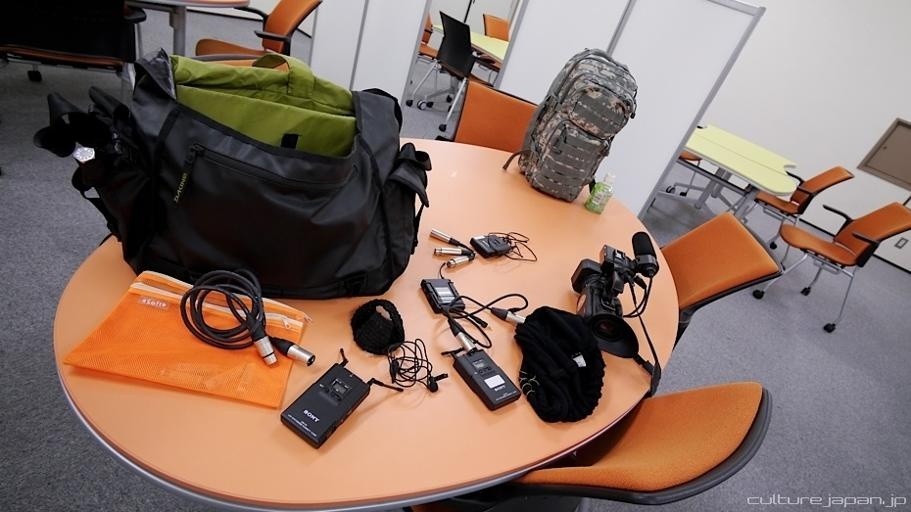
[517, 44, 641, 206]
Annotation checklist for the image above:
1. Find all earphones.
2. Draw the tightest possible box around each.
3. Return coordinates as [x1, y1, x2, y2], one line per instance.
[392, 360, 399, 378]
[427, 374, 438, 392]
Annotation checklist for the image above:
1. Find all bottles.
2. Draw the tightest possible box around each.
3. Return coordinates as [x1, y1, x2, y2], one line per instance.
[584, 169, 617, 215]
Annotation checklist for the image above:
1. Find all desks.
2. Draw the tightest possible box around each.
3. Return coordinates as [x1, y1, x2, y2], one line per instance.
[54, 138, 680, 511]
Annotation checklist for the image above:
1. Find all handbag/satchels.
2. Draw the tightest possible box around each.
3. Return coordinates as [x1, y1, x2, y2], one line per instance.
[35, 43, 432, 307]
